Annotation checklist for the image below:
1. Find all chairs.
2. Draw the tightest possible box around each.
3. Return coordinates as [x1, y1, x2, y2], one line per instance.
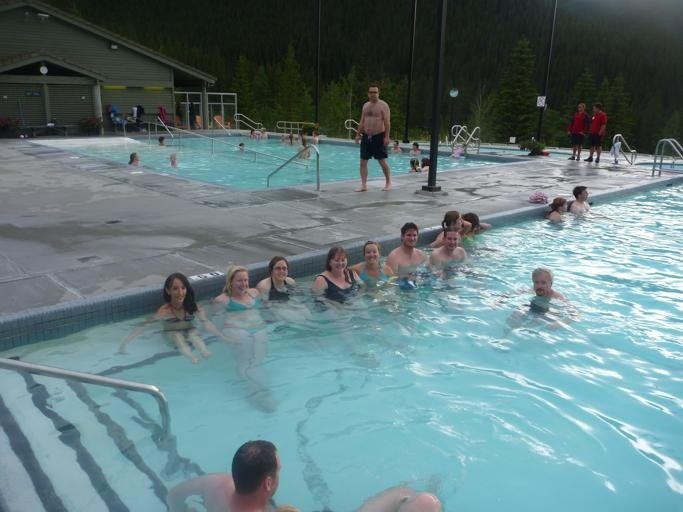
[195, 115, 213, 129]
[214, 115, 231, 130]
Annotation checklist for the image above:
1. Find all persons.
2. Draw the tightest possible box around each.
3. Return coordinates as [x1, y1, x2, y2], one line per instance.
[214, 265, 259, 301]
[0, 115, 28, 138]
[355, 85, 392, 191]
[391, 141, 467, 173]
[250, 128, 319, 159]
[239, 143, 245, 150]
[545, 186, 590, 221]
[128, 152, 139, 166]
[312, 211, 491, 291]
[170, 153, 177, 168]
[166, 440, 441, 512]
[157, 272, 201, 316]
[531, 267, 564, 298]
[584, 104, 607, 163]
[159, 137, 164, 145]
[256, 256, 296, 288]
[566, 102, 591, 160]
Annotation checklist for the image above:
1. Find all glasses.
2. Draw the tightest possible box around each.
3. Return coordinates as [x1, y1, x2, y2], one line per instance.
[369, 91, 377, 93]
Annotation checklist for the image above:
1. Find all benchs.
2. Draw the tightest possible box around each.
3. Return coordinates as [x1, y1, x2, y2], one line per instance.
[17, 125, 97, 138]
[114, 113, 174, 135]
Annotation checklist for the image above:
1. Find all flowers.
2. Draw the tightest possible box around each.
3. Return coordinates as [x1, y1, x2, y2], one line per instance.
[0, 115, 24, 129]
[80, 116, 104, 129]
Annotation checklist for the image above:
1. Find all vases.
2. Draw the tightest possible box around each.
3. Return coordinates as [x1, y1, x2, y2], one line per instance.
[88, 128, 99, 137]
[7, 128, 16, 138]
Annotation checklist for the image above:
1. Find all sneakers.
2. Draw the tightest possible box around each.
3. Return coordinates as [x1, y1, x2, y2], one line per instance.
[595, 158, 599, 162]
[584, 157, 593, 162]
[568, 157, 575, 160]
[576, 157, 580, 160]
